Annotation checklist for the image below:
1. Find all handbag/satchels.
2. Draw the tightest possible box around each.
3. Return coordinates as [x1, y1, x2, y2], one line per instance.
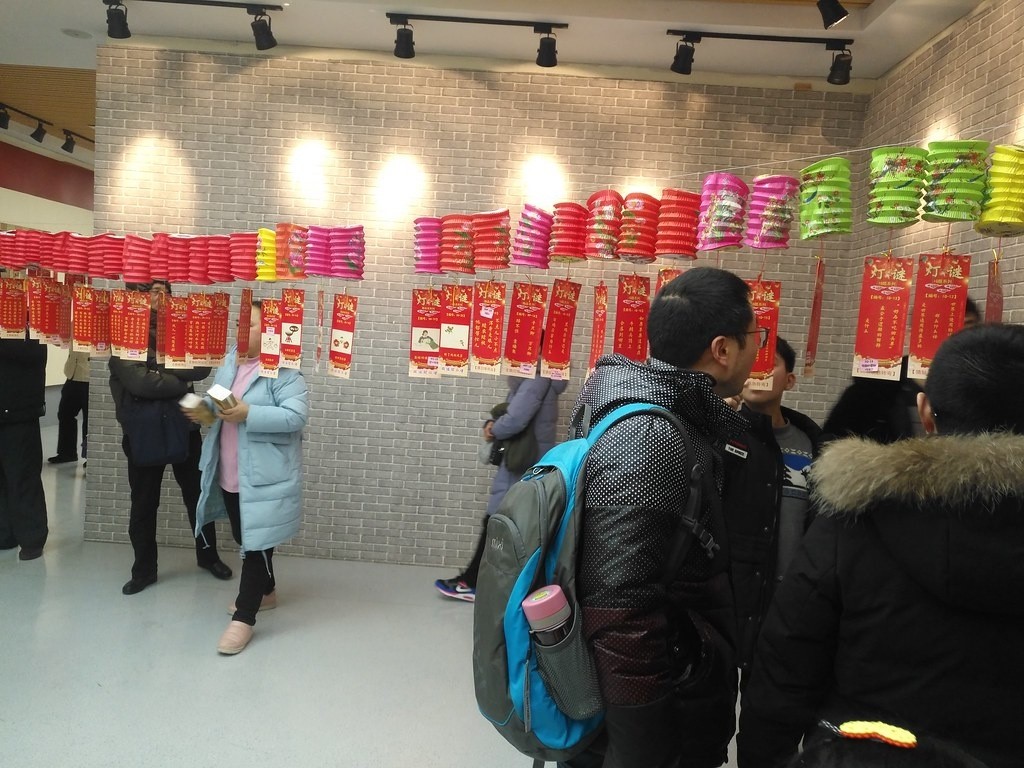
[478, 438, 506, 466]
[119, 392, 191, 466]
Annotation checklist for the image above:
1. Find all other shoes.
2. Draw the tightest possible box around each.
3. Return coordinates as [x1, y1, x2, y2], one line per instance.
[226, 589, 276, 615]
[18, 545, 43, 560]
[219, 620, 254, 655]
[0, 536, 17, 550]
[47, 452, 78, 464]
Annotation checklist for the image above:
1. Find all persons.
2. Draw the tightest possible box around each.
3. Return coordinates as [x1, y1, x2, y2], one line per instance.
[720, 330, 823, 768]
[0, 306, 48, 561]
[435, 325, 569, 605]
[104, 278, 233, 594]
[553, 267, 757, 768]
[45, 318, 89, 476]
[821, 283, 984, 452]
[191, 301, 310, 656]
[761, 316, 1024, 768]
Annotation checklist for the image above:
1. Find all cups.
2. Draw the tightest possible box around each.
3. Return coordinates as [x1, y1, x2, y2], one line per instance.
[521, 584, 601, 720]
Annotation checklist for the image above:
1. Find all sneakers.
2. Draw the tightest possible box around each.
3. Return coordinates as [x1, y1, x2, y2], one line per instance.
[435, 575, 476, 603]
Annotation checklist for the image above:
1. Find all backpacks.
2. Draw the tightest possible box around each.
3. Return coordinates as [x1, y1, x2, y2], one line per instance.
[474, 404, 703, 762]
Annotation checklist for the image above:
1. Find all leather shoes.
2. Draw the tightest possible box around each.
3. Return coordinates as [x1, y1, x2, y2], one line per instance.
[197, 558, 233, 579]
[122, 571, 157, 594]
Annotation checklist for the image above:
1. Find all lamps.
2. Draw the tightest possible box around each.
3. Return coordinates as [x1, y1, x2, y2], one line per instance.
[61, 129, 76, 153]
[825, 41, 853, 85]
[817, 0, 849, 30]
[29, 120, 47, 143]
[103, 0, 131, 39]
[247, 6, 277, 51]
[670, 34, 702, 75]
[389, 16, 416, 58]
[0, 103, 11, 130]
[533, 24, 558, 67]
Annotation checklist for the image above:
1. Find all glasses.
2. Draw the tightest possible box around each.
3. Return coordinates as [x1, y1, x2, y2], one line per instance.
[708, 327, 770, 350]
